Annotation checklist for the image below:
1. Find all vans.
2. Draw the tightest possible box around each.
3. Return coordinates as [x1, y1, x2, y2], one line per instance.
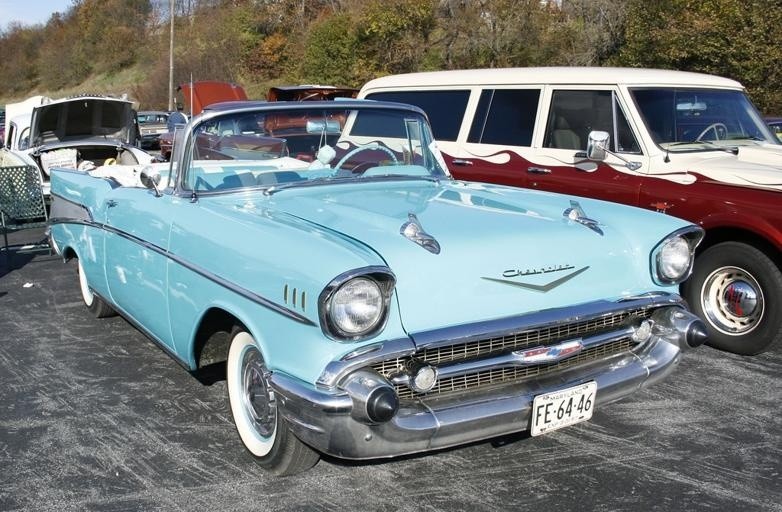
[331, 66, 782, 357]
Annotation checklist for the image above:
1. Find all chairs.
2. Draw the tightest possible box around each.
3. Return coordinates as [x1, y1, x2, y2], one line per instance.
[551, 114, 588, 150]
[0, 164, 54, 269]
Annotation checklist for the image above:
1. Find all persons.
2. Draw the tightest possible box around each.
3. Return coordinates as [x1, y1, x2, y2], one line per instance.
[166, 104, 189, 131]
